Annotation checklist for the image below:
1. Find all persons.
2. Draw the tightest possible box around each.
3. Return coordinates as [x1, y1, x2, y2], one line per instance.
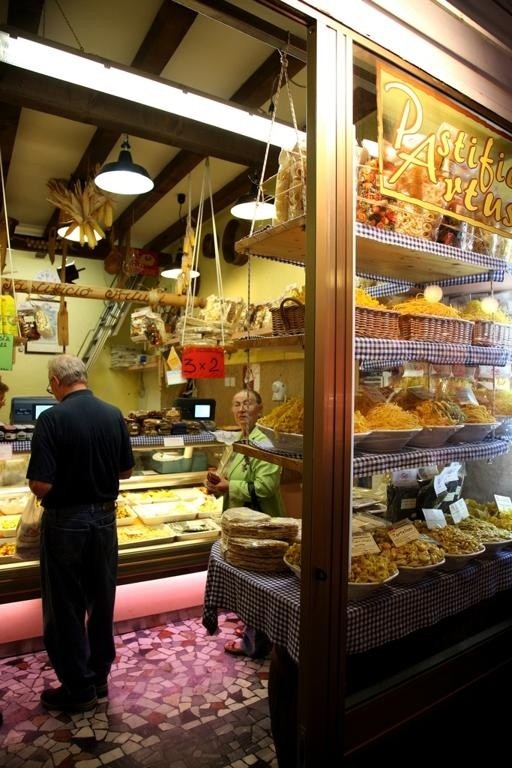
[203, 388, 287, 661]
[25, 354, 137, 714]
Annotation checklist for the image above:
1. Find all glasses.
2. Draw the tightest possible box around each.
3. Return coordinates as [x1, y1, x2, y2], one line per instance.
[46, 379, 54, 394]
[0, 399, 6, 404]
[231, 401, 248, 412]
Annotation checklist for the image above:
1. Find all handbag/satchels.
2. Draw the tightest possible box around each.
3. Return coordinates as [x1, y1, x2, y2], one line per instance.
[243, 482, 264, 513]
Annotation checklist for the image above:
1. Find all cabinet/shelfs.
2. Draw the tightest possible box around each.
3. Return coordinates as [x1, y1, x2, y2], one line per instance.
[0, 441, 223, 604]
[201, 213, 511, 679]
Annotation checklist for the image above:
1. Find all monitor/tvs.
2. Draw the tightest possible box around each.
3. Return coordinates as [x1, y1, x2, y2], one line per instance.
[194, 404, 211, 417]
[32, 403, 56, 420]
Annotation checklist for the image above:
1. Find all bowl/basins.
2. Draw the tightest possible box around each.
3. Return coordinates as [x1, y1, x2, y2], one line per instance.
[118, 523, 176, 546]
[365, 430, 423, 451]
[1, 515, 22, 537]
[117, 506, 137, 526]
[257, 421, 371, 452]
[447, 546, 487, 564]
[166, 519, 222, 540]
[411, 425, 466, 448]
[0, 538, 19, 563]
[133, 503, 198, 525]
[484, 538, 511, 553]
[453, 424, 502, 443]
[398, 559, 447, 581]
[283, 553, 399, 601]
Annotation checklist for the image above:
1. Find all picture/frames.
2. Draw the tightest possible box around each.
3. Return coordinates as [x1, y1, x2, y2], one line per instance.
[24, 298, 66, 354]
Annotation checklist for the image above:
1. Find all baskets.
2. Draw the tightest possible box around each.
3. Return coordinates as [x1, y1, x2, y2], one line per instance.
[398, 312, 476, 345]
[469, 319, 512, 347]
[269, 298, 401, 340]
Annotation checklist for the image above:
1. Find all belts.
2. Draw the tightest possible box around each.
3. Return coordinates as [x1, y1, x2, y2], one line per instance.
[76, 502, 115, 511]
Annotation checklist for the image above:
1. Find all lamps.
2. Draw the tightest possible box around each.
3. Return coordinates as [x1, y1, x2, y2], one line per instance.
[230, 168, 276, 220]
[161, 193, 199, 279]
[56, 225, 103, 242]
[93, 132, 154, 195]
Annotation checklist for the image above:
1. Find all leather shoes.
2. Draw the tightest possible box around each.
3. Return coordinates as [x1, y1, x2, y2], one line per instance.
[234, 625, 245, 638]
[224, 641, 245, 656]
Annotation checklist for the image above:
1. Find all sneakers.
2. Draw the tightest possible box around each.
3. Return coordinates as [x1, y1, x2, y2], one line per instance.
[96, 682, 108, 698]
[40, 686, 98, 712]
